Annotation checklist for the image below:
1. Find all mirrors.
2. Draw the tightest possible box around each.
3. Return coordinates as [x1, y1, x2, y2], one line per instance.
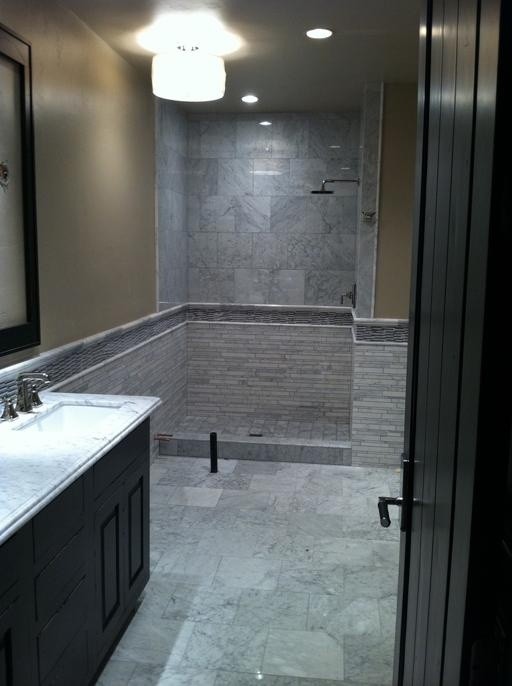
[0, 25, 41, 357]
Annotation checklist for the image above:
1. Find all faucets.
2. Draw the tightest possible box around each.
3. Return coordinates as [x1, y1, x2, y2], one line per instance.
[13, 371, 51, 411]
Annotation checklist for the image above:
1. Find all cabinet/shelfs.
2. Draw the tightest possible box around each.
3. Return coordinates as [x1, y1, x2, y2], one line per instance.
[98, 415, 152, 665]
[1, 466, 100, 686]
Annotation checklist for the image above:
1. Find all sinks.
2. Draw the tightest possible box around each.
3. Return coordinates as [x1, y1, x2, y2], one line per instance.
[12, 401, 124, 434]
